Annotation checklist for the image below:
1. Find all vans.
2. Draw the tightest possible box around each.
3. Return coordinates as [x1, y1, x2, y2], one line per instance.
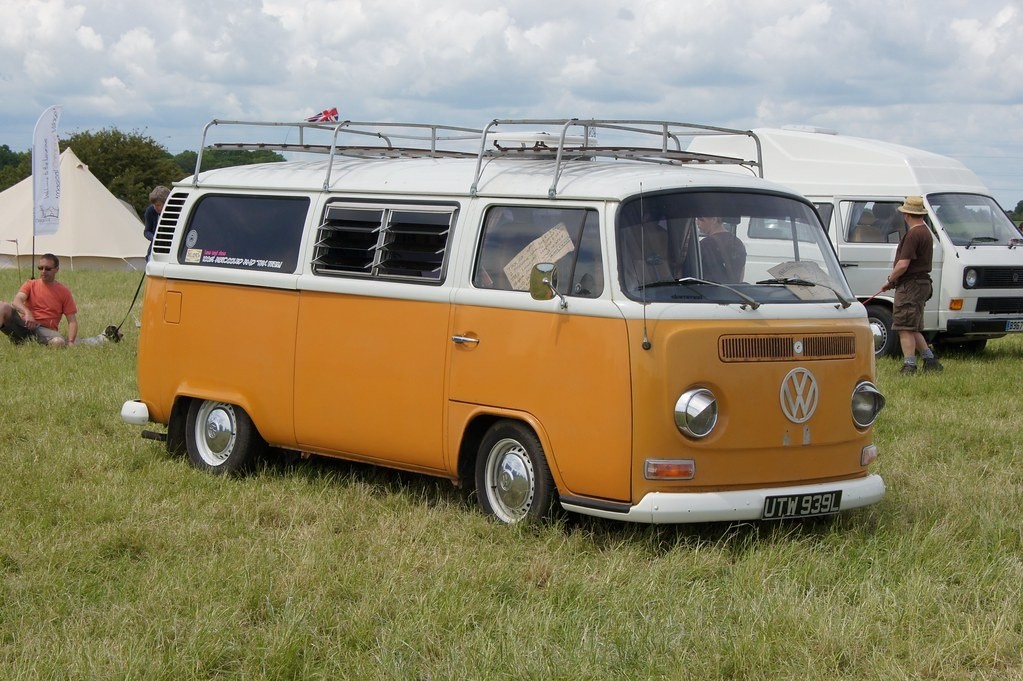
[120, 117, 888, 535]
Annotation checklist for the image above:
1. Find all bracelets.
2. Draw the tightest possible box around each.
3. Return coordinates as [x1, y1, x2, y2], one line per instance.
[888, 276, 890, 282]
[69, 340, 73, 342]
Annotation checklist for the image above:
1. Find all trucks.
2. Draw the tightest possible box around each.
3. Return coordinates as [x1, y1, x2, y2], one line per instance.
[683, 126, 1023, 360]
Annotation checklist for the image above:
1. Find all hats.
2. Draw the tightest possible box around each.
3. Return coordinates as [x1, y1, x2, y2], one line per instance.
[898, 196, 928, 215]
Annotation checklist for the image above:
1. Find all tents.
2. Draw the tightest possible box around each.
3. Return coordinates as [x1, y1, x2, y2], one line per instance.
[1, 146, 151, 269]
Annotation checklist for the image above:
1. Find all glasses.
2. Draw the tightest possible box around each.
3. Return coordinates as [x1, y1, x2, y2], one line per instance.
[38, 265, 56, 271]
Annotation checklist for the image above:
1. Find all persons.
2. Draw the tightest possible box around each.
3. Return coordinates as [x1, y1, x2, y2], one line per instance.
[0, 254, 78, 348]
[144, 185, 170, 262]
[684, 216, 747, 284]
[881, 197, 943, 373]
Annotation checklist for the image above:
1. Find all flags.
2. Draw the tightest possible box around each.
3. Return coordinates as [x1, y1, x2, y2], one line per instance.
[308, 107, 338, 123]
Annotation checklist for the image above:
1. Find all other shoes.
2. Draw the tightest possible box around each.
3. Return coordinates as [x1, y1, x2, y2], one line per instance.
[922, 355, 944, 375]
[898, 362, 917, 375]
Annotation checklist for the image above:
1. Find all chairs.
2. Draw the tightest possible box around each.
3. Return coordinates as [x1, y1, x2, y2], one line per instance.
[624, 224, 676, 284]
[850, 211, 883, 243]
